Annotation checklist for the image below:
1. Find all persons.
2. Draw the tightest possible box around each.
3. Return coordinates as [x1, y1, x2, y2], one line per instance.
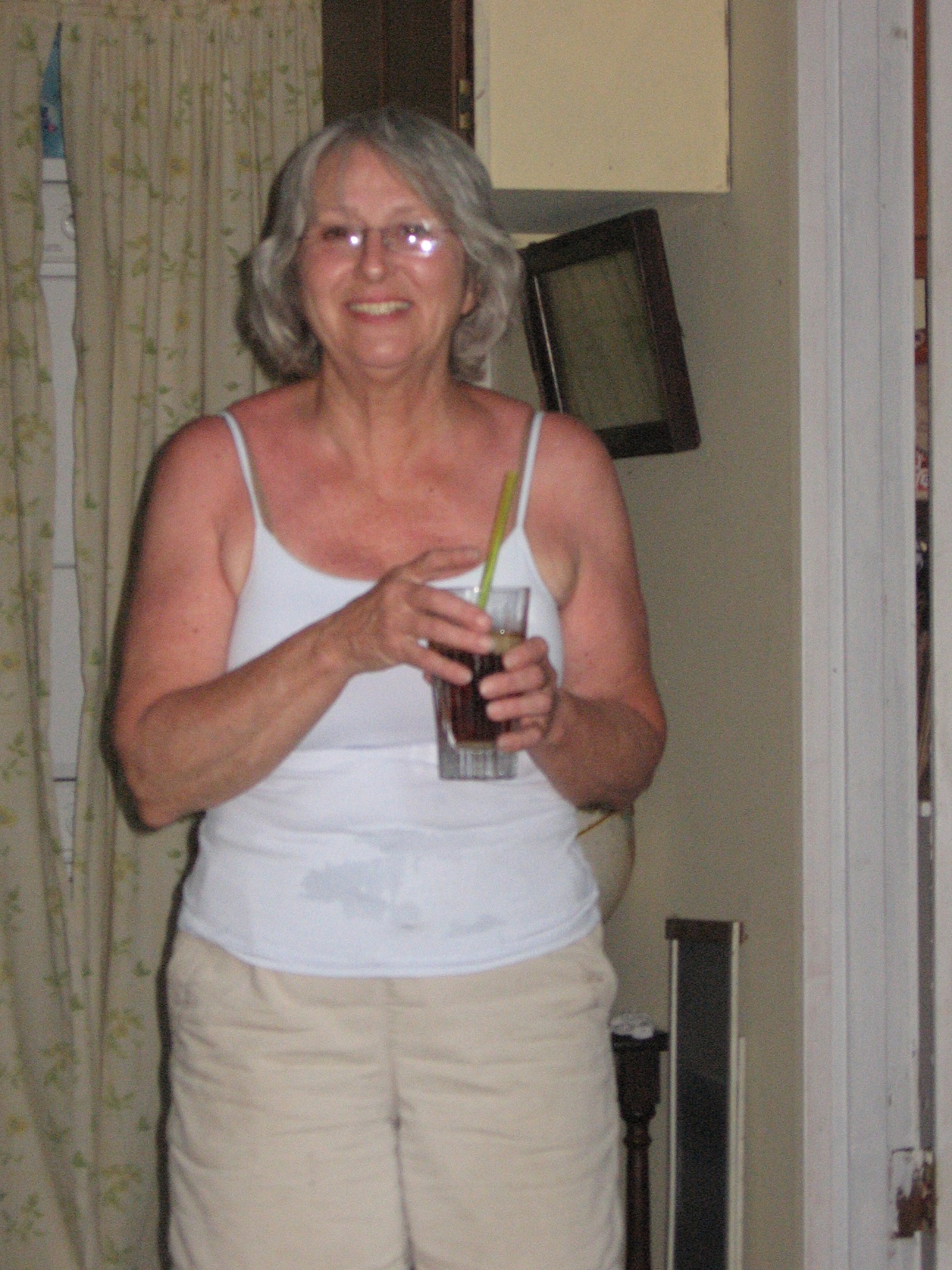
[112, 115, 667, 1270]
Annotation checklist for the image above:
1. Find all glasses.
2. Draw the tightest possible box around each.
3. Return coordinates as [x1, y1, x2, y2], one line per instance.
[299, 218, 454, 260]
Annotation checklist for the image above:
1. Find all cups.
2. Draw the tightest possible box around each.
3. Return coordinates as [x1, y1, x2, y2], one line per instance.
[427, 582, 531, 780]
